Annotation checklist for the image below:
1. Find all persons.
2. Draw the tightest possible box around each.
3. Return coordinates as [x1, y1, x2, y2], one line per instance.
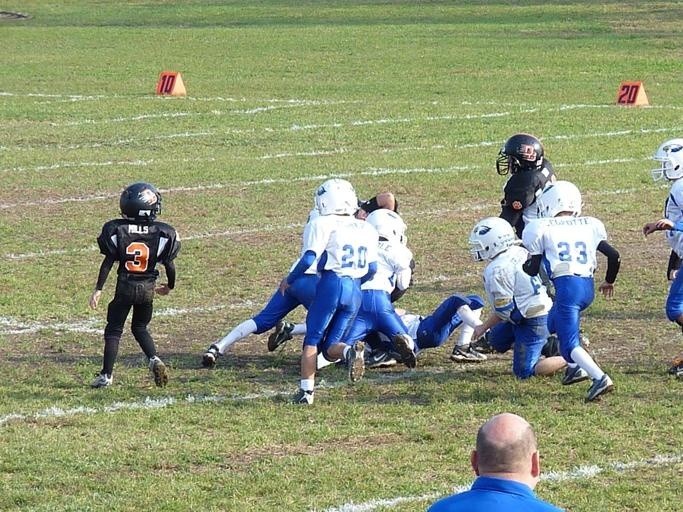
[495, 135, 557, 239]
[469, 216, 590, 384]
[522, 179, 620, 402]
[644, 140, 683, 377]
[426, 412, 568, 512]
[87, 183, 181, 388]
[202, 178, 416, 401]
[365, 293, 491, 363]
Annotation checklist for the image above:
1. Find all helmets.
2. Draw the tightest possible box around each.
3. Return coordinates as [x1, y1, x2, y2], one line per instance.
[365, 208, 408, 246]
[535, 180, 583, 224]
[119, 183, 162, 222]
[467, 216, 516, 262]
[650, 138, 683, 183]
[315, 178, 359, 216]
[496, 133, 545, 177]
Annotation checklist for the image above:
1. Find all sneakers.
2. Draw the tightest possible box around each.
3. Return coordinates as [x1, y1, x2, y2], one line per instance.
[345, 339, 366, 383]
[364, 351, 397, 369]
[91, 370, 113, 389]
[586, 371, 615, 402]
[202, 344, 223, 368]
[285, 387, 315, 407]
[561, 365, 588, 385]
[450, 342, 488, 363]
[267, 319, 296, 352]
[468, 336, 493, 354]
[147, 355, 169, 388]
[393, 333, 417, 369]
